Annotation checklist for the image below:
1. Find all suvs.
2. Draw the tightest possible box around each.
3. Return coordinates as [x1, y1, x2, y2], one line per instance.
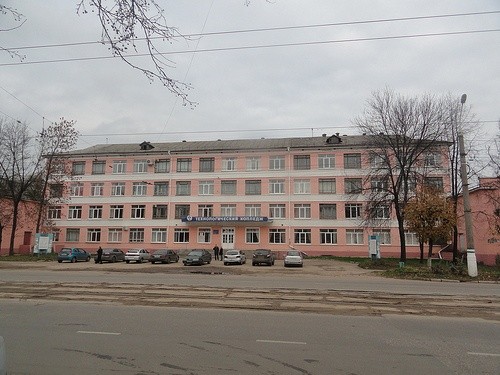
[251, 248, 275, 266]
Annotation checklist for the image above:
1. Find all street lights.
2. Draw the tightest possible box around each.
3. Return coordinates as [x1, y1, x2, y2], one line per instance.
[457, 93, 479, 277]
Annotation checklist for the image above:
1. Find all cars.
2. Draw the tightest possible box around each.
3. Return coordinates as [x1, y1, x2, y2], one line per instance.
[150, 249, 180, 264]
[124, 247, 152, 264]
[182, 248, 212, 266]
[57, 247, 92, 263]
[223, 249, 247, 266]
[94, 247, 125, 263]
[282, 249, 305, 267]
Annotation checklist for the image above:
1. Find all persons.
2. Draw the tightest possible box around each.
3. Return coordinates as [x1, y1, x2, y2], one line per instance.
[212, 244, 223, 261]
[95, 246, 103, 264]
